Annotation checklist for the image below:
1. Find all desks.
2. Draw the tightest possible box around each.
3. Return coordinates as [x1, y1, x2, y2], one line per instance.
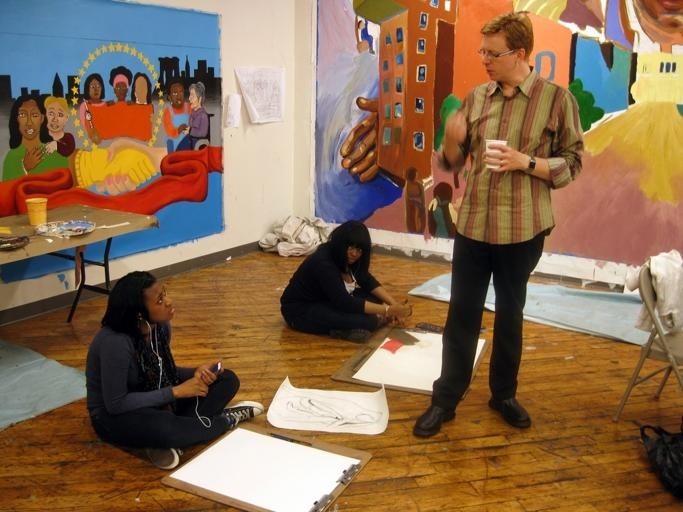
[2, 204, 156, 324]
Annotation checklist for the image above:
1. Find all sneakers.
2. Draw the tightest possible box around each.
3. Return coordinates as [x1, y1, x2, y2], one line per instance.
[330, 328, 370, 342]
[219, 400, 264, 428]
[146, 447, 179, 470]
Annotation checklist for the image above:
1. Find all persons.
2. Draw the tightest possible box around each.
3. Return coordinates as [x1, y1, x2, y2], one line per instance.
[2, 94, 68, 182]
[280, 220, 414, 343]
[176, 82, 210, 149]
[131, 70, 152, 105]
[86, 272, 265, 470]
[44, 95, 75, 158]
[414, 11, 585, 436]
[79, 72, 106, 125]
[107, 65, 132, 108]
[163, 79, 191, 155]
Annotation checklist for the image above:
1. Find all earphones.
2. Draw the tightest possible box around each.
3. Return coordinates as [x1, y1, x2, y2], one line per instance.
[138, 315, 212, 429]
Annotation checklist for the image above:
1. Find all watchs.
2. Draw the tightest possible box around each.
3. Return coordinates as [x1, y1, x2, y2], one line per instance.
[524, 154, 537, 174]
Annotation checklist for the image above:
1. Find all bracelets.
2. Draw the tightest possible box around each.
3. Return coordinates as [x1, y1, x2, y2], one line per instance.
[385, 304, 390, 320]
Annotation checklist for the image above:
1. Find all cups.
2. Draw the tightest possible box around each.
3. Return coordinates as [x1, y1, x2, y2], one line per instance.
[25, 198, 47, 225]
[485, 140, 507, 168]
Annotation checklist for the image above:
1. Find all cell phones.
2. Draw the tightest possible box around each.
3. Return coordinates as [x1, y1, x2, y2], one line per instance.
[209, 360, 222, 373]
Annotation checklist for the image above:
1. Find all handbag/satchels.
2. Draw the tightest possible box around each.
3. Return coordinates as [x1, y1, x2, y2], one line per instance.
[638, 422, 683, 500]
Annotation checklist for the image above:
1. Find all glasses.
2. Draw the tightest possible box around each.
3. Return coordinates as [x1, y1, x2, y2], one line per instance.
[476, 48, 516, 58]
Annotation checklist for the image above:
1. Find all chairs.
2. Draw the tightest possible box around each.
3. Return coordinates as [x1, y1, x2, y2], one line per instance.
[612, 256, 681, 424]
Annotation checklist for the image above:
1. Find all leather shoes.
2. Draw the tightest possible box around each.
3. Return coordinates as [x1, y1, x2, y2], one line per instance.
[413, 404, 456, 437]
[489, 396, 531, 427]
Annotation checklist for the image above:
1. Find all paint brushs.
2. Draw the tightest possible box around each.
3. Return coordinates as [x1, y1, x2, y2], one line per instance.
[266, 431, 312, 448]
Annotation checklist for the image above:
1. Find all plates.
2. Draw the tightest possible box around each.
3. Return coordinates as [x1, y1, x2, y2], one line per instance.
[35, 221, 95, 237]
